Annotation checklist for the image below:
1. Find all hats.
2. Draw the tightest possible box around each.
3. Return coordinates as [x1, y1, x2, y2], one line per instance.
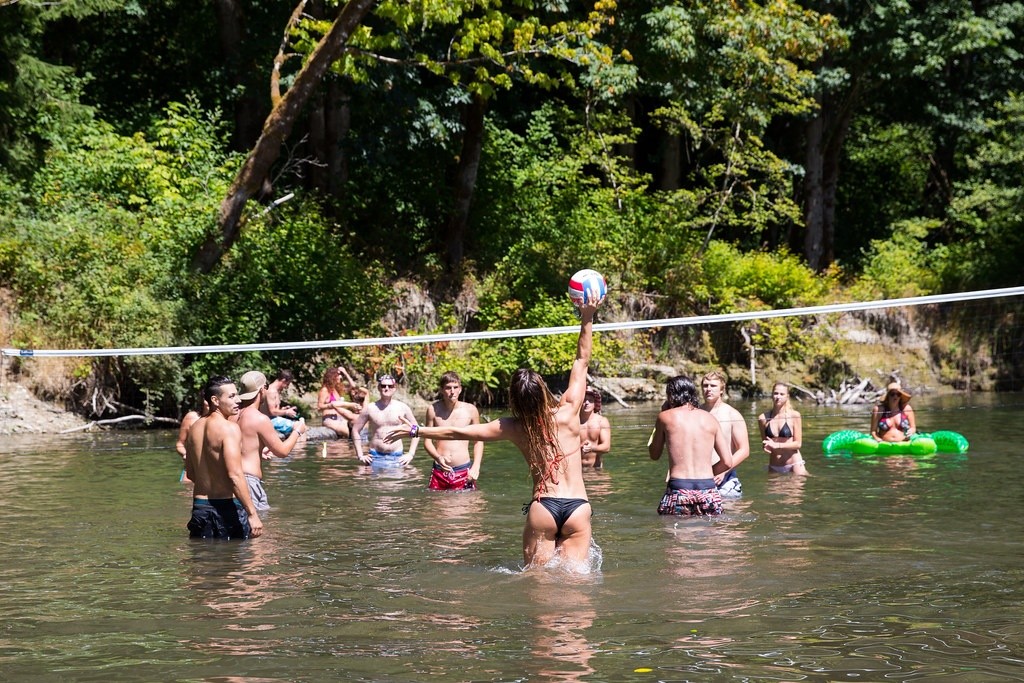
[878, 382, 912, 404]
[238, 371, 266, 400]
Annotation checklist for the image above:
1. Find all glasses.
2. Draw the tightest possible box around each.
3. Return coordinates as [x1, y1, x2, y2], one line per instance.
[888, 392, 902, 397]
[380, 384, 395, 388]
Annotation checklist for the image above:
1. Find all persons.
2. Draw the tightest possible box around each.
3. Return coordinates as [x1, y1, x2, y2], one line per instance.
[383, 288, 606, 571]
[576, 390, 610, 468]
[871, 383, 916, 444]
[649, 370, 750, 514]
[757, 383, 808, 475]
[176, 365, 420, 540]
[423, 370, 484, 491]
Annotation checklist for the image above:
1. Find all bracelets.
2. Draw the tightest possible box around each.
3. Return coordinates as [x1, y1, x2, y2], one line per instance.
[294, 428, 302, 436]
[409, 425, 419, 438]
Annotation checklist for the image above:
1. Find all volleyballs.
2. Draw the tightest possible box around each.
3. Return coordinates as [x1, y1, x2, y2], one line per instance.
[568, 269, 607, 308]
